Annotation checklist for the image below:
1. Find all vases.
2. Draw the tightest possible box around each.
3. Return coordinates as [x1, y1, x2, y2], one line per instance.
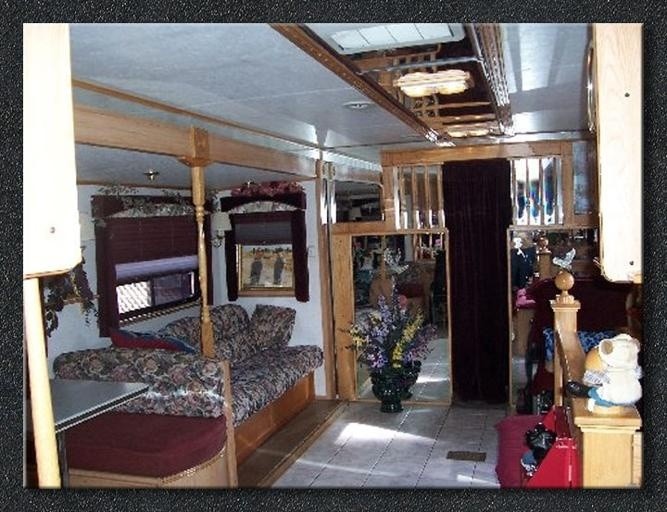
[400, 360, 419, 400]
[370, 369, 406, 412]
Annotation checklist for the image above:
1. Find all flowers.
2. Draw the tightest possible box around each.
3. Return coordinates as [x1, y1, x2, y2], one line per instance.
[402, 284, 438, 365]
[45, 265, 103, 335]
[336, 289, 427, 371]
[232, 181, 306, 197]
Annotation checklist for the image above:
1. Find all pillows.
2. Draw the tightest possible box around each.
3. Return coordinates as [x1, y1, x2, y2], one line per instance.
[250, 304, 296, 348]
[111, 327, 197, 354]
[543, 326, 617, 362]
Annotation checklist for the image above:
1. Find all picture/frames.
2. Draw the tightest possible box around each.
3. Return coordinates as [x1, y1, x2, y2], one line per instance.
[237, 243, 296, 296]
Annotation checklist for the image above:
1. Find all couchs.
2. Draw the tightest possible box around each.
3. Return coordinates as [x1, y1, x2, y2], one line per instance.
[524, 275, 604, 385]
[53, 345, 239, 489]
[528, 277, 642, 415]
[110, 304, 324, 468]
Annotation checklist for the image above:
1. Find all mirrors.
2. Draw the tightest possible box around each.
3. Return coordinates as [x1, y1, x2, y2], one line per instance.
[350, 231, 450, 402]
[267, 22, 514, 147]
[506, 224, 599, 416]
